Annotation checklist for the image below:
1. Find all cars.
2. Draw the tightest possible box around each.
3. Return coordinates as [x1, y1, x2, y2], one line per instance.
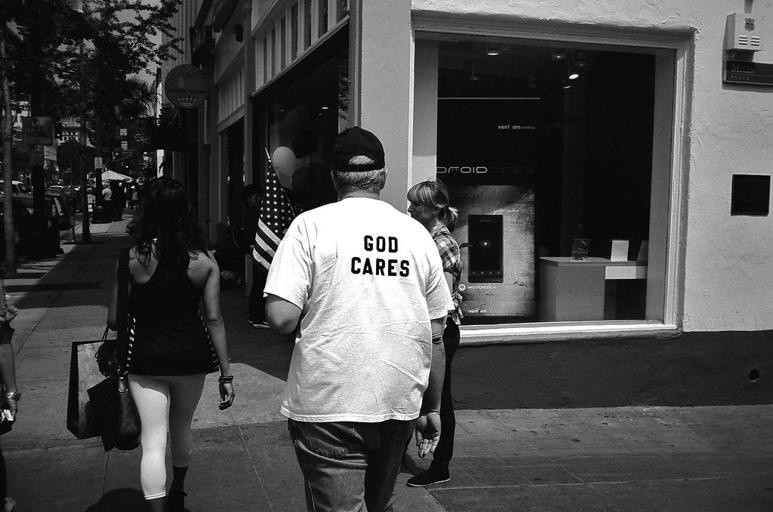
[1, 181, 81, 258]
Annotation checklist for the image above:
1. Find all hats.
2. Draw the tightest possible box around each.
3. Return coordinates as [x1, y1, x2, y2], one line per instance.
[329, 126, 384, 172]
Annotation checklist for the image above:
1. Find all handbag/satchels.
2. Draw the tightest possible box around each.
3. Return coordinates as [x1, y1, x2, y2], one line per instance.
[66, 341, 116, 440]
[231, 224, 252, 254]
[97, 337, 135, 376]
[112, 372, 141, 450]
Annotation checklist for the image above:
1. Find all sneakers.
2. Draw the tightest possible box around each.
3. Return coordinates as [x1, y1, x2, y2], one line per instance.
[407, 467, 451, 487]
[247, 316, 271, 329]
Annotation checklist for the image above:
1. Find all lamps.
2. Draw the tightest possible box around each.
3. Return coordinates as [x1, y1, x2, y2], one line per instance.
[547, 61, 582, 82]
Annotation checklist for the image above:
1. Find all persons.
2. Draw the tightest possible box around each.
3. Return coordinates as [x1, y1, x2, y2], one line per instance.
[262, 126, 456, 511]
[107, 175, 237, 511]
[0, 278, 20, 511]
[101, 176, 147, 220]
[398, 181, 463, 488]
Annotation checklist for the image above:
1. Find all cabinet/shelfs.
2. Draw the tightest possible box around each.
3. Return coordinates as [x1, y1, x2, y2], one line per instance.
[540, 255, 647, 320]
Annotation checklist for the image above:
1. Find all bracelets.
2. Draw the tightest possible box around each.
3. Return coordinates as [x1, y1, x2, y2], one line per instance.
[220, 374, 234, 385]
[4, 392, 19, 401]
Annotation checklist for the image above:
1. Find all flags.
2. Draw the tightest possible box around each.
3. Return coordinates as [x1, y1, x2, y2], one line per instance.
[247, 164, 298, 329]
